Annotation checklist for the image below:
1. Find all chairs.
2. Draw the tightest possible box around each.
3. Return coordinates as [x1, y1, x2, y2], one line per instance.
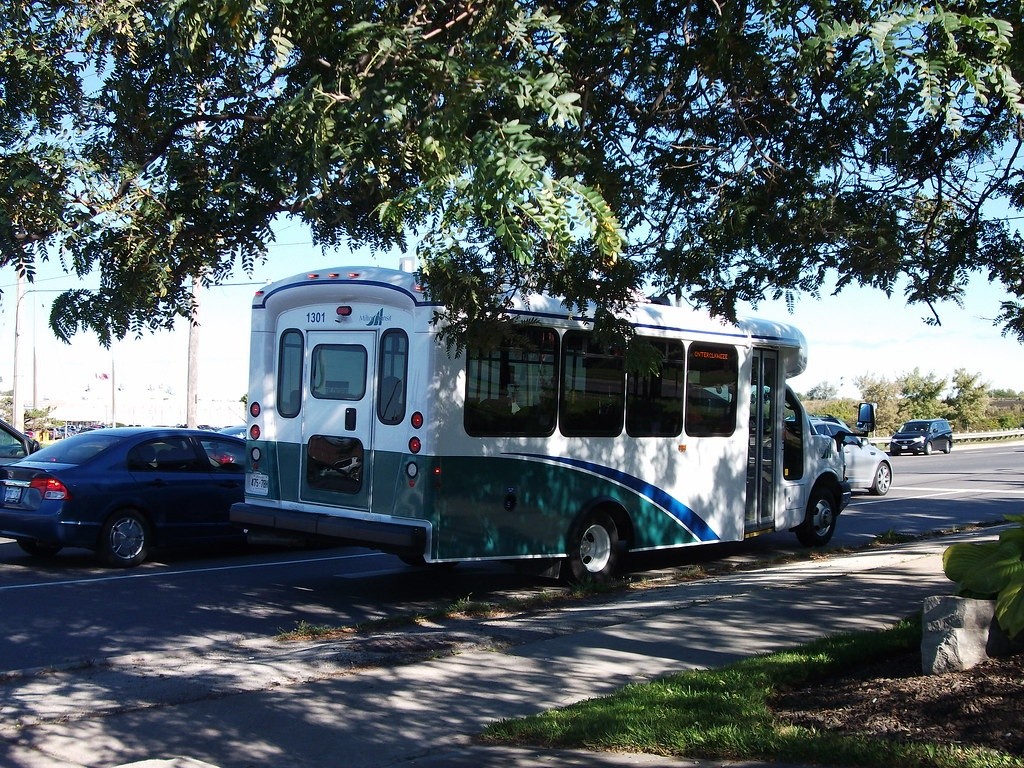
[137, 445, 189, 471]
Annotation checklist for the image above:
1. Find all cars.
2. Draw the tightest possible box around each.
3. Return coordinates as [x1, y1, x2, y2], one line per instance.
[218, 425, 249, 439]
[811, 419, 893, 498]
[0, 427, 252, 566]
[0, 417, 48, 480]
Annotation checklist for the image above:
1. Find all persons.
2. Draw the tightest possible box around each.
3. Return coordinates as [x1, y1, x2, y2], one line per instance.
[381, 377, 402, 420]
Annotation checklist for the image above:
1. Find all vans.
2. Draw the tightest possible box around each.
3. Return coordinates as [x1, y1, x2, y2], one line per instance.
[890, 418, 953, 456]
[229, 268, 877, 591]
